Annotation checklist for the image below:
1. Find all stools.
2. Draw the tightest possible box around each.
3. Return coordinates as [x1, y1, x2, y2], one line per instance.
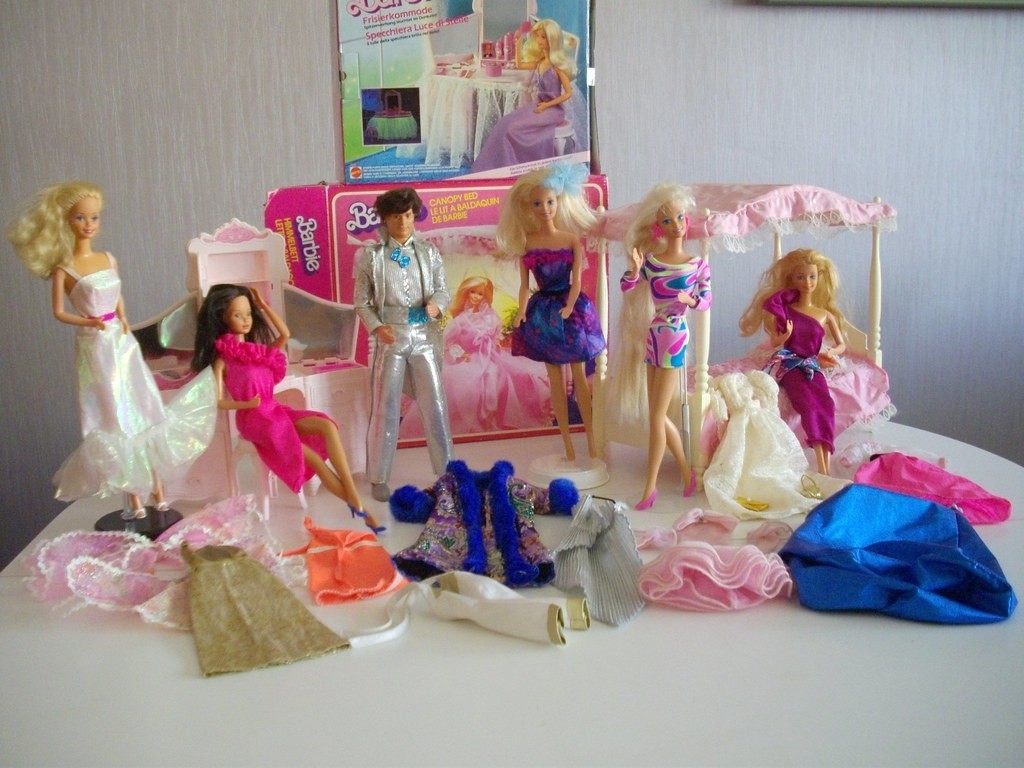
[553, 118, 580, 158]
[230, 436, 309, 520]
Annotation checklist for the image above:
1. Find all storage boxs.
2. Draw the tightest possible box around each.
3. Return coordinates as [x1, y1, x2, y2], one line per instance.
[264, 172, 608, 451]
[336, 0, 601, 183]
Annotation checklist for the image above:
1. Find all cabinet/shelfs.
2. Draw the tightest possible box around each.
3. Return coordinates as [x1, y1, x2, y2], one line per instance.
[130, 217, 370, 496]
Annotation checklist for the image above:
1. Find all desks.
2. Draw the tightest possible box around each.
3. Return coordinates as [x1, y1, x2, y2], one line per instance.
[398, 74, 532, 169]
[0, 420, 1024, 768]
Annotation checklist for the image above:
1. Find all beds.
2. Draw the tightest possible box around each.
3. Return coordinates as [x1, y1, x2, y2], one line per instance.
[580, 184, 897, 493]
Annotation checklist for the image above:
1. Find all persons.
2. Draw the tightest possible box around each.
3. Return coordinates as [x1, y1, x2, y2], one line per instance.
[620, 183, 713, 509]
[6, 179, 170, 520]
[490, 166, 606, 464]
[468, 18, 581, 173]
[738, 248, 850, 476]
[190, 284, 387, 536]
[354, 184, 455, 502]
